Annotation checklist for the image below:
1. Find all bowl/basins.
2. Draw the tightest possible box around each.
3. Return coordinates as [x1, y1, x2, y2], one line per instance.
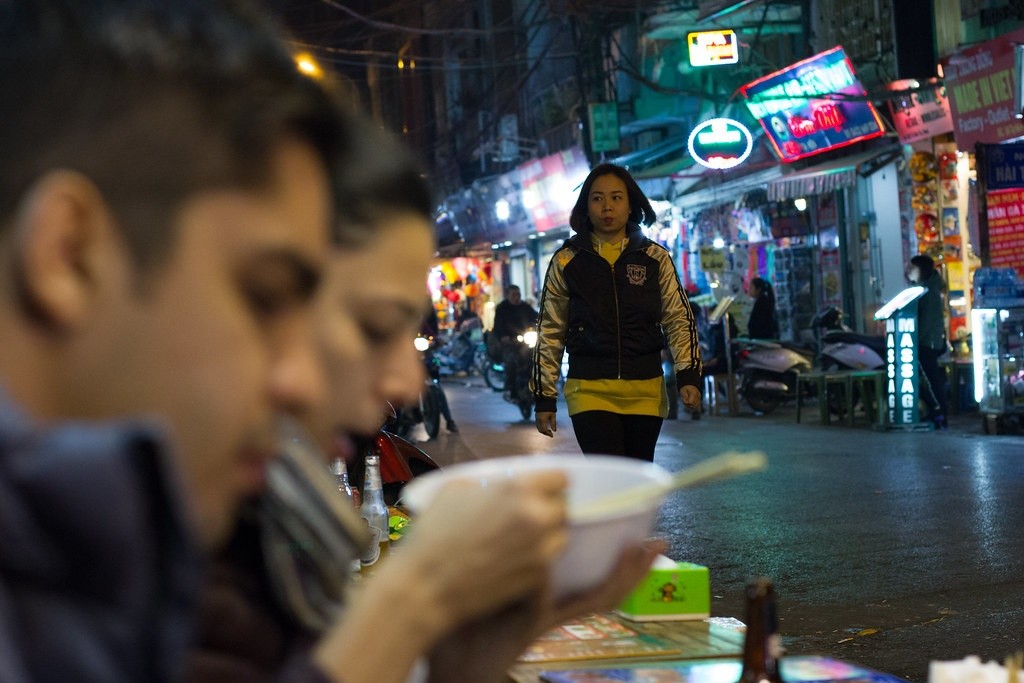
[399, 452, 673, 600]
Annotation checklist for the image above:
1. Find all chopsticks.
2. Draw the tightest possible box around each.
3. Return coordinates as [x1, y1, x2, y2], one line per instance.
[565, 447, 765, 517]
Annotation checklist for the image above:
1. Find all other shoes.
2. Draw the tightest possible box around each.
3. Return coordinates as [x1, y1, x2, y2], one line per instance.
[691, 408, 701, 420]
[447, 420, 457, 432]
[920, 409, 947, 423]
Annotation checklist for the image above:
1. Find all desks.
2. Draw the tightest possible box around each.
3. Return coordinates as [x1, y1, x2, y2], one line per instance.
[361, 503, 889, 683]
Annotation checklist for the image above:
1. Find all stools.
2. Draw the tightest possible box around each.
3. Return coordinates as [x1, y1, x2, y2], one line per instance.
[795, 372, 885, 427]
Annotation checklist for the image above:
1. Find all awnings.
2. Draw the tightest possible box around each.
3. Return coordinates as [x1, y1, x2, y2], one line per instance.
[766, 144, 898, 201]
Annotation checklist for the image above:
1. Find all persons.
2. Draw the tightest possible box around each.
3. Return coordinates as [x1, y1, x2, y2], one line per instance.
[406, 404, 429, 443]
[676, 300, 739, 420]
[0, 1, 668, 683]
[416, 307, 459, 433]
[180, 103, 436, 683]
[905, 254, 948, 425]
[745, 278, 781, 342]
[529, 163, 701, 463]
[490, 285, 539, 402]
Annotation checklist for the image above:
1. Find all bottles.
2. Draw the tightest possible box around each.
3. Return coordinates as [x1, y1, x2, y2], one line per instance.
[328, 454, 355, 516]
[733, 577, 789, 683]
[358, 455, 392, 576]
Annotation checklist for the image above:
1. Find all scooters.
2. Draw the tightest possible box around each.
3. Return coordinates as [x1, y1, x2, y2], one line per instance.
[435, 329, 537, 420]
[410, 333, 448, 438]
[810, 330, 886, 413]
[728, 306, 854, 416]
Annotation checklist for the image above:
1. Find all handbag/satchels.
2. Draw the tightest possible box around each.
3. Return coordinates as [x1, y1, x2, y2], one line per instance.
[937, 337, 953, 359]
